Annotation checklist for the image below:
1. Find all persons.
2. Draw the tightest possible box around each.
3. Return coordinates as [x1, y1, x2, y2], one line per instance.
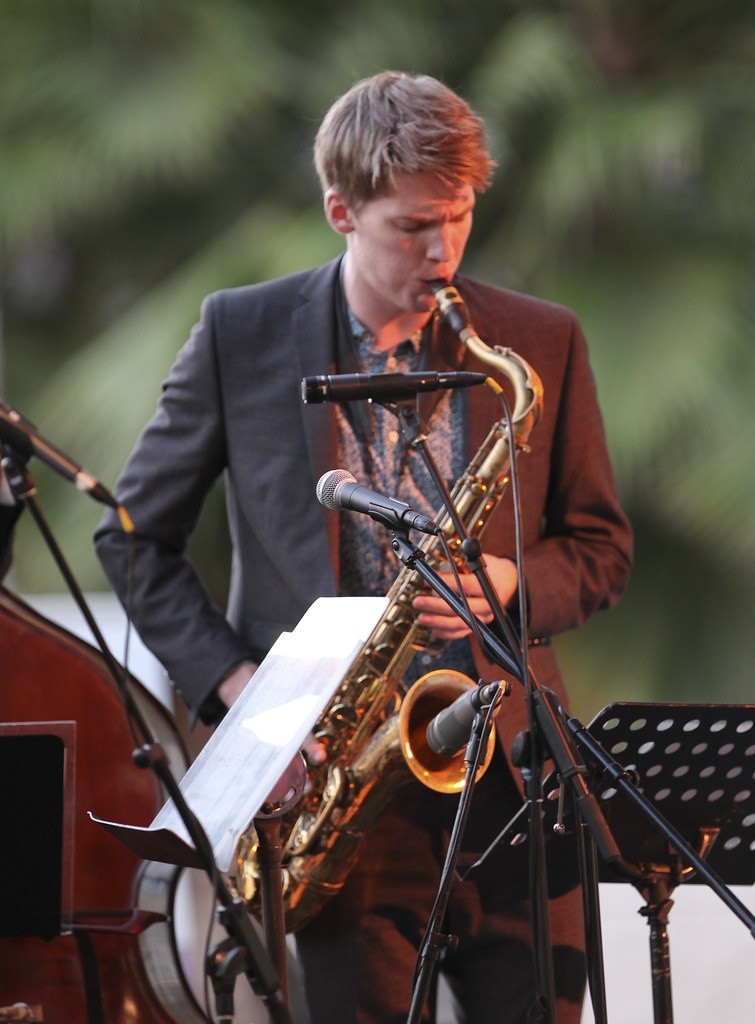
[92, 69, 635, 1024]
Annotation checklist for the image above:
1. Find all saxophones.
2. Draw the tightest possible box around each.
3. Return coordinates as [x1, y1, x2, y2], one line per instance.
[237, 280, 546, 925]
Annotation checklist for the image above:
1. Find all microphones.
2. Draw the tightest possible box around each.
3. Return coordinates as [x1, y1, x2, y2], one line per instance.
[0, 401, 115, 510]
[300, 371, 486, 405]
[316, 469, 442, 535]
[425, 679, 509, 757]
